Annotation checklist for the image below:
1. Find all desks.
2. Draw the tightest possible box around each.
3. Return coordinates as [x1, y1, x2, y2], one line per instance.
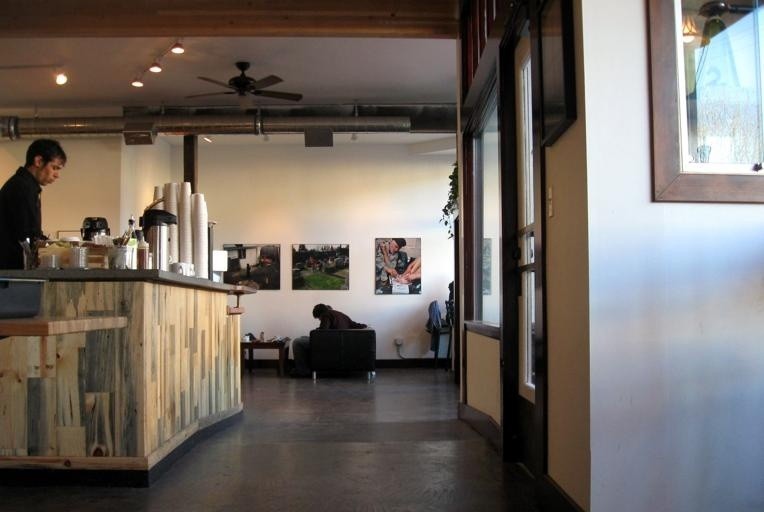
[0, 313, 130, 340]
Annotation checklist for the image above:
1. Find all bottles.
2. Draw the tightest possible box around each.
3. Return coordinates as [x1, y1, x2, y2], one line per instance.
[259, 328, 264, 342]
[135, 237, 149, 270]
[128, 219, 135, 237]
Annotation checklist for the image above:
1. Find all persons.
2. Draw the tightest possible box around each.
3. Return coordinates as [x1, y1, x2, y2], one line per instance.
[257, 247, 279, 290]
[0, 138, 68, 269]
[376, 238, 420, 289]
[292, 303, 366, 378]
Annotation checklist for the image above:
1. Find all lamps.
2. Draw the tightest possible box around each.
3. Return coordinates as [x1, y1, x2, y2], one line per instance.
[129, 36, 184, 88]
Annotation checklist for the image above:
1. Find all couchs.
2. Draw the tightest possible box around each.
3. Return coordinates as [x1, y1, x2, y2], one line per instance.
[309, 328, 376, 384]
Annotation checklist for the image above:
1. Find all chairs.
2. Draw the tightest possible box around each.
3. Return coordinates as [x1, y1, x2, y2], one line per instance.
[429, 299, 455, 372]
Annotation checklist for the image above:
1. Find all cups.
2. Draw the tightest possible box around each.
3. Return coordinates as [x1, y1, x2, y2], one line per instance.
[242, 335, 249, 342]
[153, 181, 209, 281]
[38, 246, 88, 270]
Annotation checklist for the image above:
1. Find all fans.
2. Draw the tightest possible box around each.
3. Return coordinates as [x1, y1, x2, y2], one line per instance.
[185, 60, 304, 102]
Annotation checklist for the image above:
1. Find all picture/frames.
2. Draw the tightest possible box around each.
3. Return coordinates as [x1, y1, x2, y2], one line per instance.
[647, 0, 762, 204]
